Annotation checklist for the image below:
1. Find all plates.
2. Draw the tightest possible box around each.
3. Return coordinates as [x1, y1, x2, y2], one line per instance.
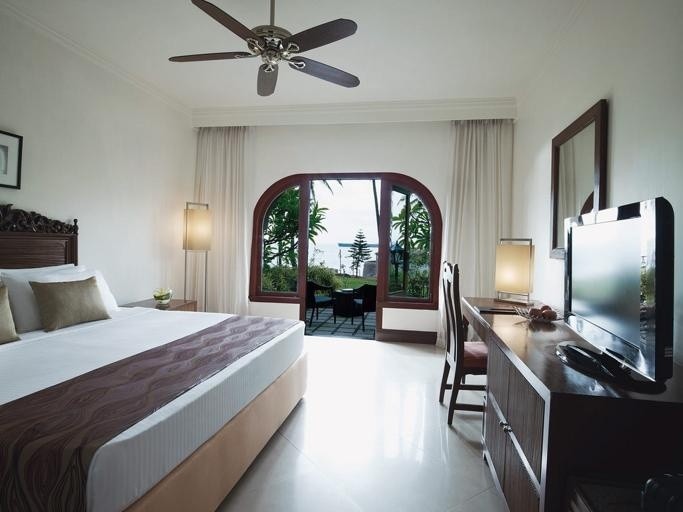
[513, 305, 564, 324]
[340, 288, 354, 292]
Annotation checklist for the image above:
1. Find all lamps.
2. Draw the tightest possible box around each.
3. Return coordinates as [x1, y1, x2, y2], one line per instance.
[493, 235, 538, 309]
[182, 201, 211, 312]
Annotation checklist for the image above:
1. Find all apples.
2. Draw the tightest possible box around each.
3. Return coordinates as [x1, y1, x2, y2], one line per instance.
[528, 305, 557, 319]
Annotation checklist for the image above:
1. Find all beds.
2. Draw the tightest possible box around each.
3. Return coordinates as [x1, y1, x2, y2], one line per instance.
[1, 201, 306, 512]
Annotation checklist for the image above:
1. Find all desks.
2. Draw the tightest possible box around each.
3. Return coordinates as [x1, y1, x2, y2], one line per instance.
[332, 287, 361, 326]
[460, 290, 682, 512]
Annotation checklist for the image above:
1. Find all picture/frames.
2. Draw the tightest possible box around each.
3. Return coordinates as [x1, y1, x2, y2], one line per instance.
[0, 129, 23, 190]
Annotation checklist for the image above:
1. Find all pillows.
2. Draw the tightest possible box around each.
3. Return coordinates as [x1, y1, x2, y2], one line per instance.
[0, 262, 75, 287]
[0, 266, 123, 334]
[28, 276, 111, 332]
[0, 283, 22, 345]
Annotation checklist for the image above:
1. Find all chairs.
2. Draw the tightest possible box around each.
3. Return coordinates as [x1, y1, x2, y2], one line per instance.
[436, 261, 487, 427]
[350, 284, 380, 333]
[304, 280, 336, 328]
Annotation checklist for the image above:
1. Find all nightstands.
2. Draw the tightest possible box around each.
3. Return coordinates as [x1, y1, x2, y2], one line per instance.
[119, 296, 197, 313]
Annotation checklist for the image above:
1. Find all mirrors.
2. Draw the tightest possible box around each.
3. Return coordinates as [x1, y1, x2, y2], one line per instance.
[547, 99, 608, 261]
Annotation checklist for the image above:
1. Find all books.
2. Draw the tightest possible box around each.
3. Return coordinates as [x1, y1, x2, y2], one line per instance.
[473, 306, 528, 315]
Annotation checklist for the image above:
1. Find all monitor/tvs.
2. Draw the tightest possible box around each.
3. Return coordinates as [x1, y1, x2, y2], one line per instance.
[555, 197, 673, 394]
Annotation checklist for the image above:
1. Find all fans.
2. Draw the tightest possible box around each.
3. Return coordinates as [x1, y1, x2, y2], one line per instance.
[167, 0, 358, 98]
